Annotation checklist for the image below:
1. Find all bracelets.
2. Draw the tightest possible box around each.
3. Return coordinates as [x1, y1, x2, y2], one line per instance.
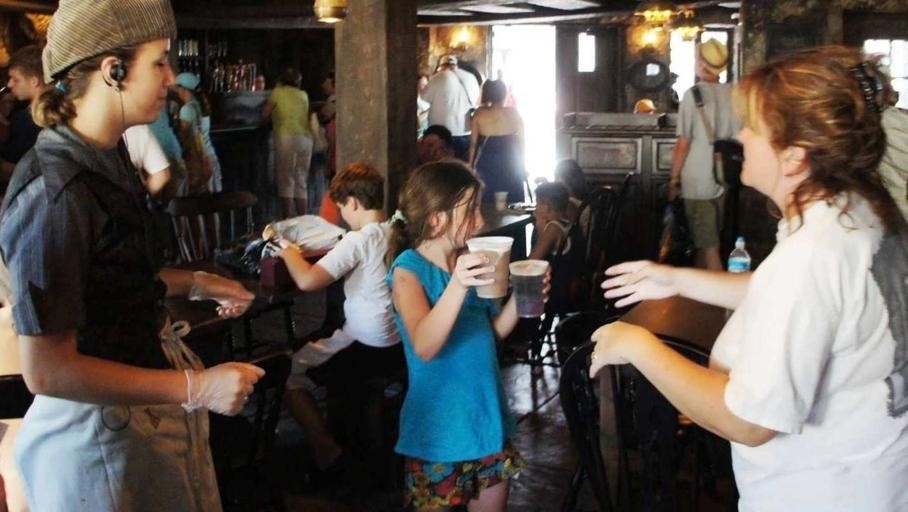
[668, 174, 681, 185]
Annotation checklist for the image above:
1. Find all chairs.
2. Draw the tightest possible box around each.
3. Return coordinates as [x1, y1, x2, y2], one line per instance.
[0, 167, 782, 512]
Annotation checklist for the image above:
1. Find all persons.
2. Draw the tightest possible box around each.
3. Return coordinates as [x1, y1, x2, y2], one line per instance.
[589, 44, 908, 512]
[669, 34, 742, 269]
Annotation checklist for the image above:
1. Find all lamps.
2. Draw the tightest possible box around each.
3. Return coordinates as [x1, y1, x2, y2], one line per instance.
[312, 0, 353, 26]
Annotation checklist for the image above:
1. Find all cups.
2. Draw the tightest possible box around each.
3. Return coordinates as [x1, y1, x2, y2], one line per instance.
[509, 260, 550, 319]
[493, 191, 509, 211]
[466, 235, 512, 299]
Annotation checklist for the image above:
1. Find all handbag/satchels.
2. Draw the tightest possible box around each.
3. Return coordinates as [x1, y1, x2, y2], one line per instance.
[185, 104, 214, 189]
[713, 139, 744, 188]
[262, 213, 346, 257]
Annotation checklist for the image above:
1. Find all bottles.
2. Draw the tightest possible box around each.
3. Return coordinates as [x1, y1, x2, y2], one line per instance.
[728, 236, 752, 273]
[176, 39, 264, 93]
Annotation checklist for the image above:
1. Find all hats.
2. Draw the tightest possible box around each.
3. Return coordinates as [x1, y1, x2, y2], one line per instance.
[37, 0, 177, 83]
[439, 54, 458, 66]
[696, 37, 730, 76]
[176, 72, 200, 91]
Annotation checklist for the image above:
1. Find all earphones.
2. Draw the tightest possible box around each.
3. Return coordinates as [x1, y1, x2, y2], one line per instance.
[110, 61, 127, 81]
